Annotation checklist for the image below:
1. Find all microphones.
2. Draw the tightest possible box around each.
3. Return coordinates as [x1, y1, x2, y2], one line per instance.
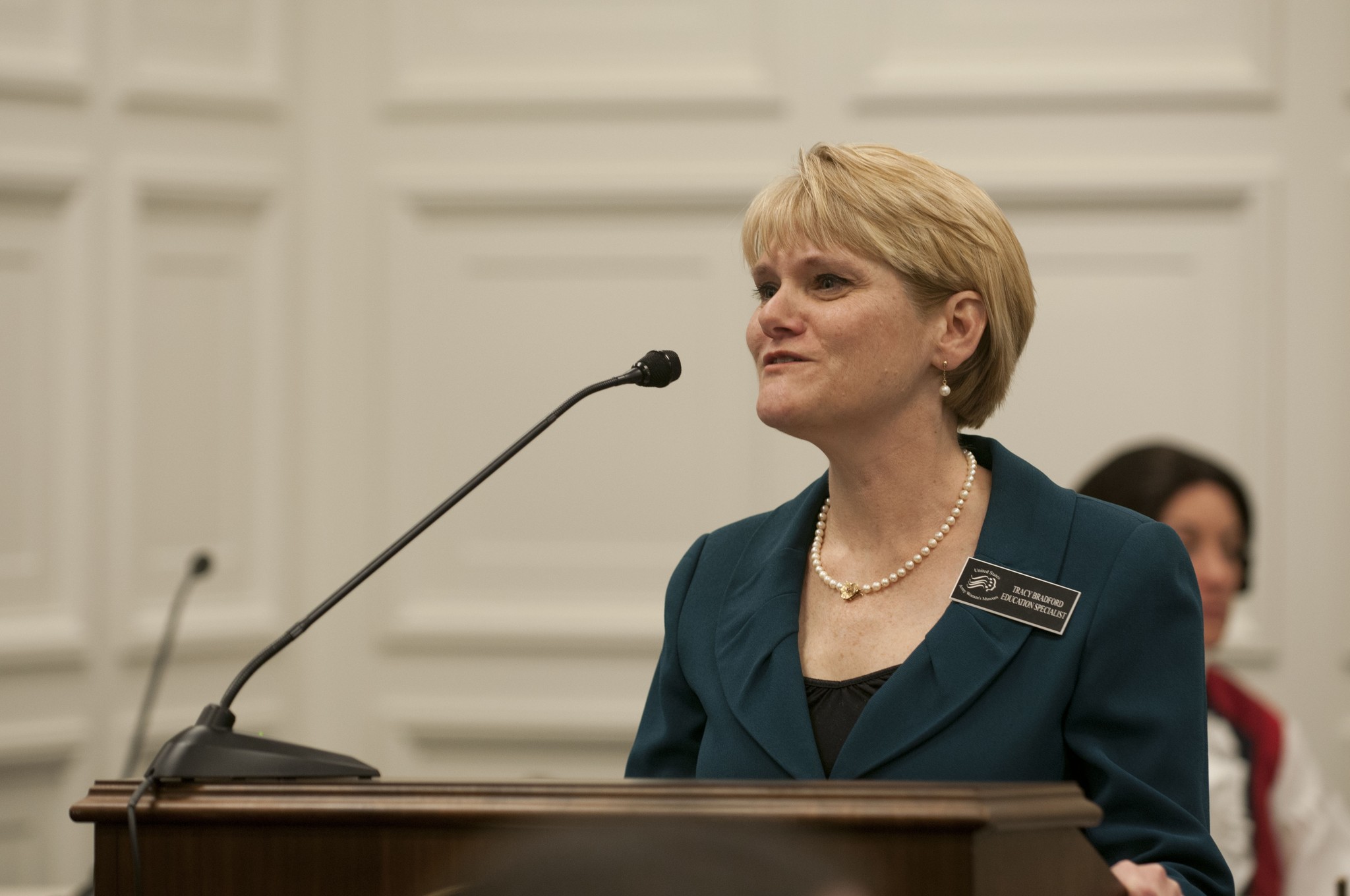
[148, 350, 682, 783]
[123, 556, 210, 777]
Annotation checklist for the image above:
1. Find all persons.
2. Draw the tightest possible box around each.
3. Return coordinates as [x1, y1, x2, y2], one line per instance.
[621, 142, 1235, 896]
[1076, 443, 1350, 896]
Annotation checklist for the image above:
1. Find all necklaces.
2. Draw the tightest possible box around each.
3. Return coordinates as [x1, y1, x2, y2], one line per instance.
[810, 447, 975, 600]
